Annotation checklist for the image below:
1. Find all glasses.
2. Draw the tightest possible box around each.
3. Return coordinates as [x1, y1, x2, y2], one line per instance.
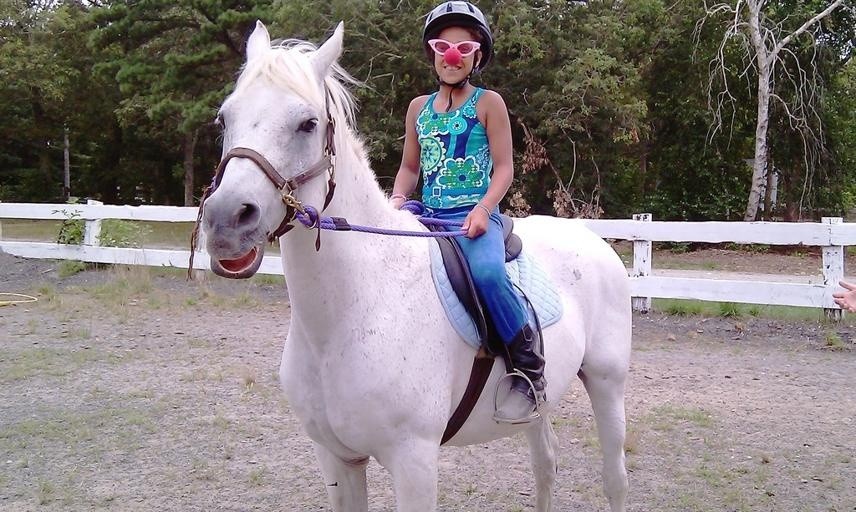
[428, 39, 481, 56]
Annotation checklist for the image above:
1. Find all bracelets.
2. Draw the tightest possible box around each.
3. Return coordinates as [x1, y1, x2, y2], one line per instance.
[474, 204, 491, 218]
[389, 193, 408, 203]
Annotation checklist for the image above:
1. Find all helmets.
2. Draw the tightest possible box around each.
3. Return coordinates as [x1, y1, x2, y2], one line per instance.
[420, 0, 495, 71]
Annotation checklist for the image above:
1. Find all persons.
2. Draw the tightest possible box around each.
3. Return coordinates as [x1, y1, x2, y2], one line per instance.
[384, 0, 548, 423]
[833, 280, 856, 313]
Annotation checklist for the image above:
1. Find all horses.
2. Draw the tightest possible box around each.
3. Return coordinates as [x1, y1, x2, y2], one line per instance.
[195, 16, 635, 511]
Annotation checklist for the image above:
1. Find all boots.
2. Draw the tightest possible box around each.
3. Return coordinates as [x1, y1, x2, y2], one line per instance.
[492, 320, 548, 424]
